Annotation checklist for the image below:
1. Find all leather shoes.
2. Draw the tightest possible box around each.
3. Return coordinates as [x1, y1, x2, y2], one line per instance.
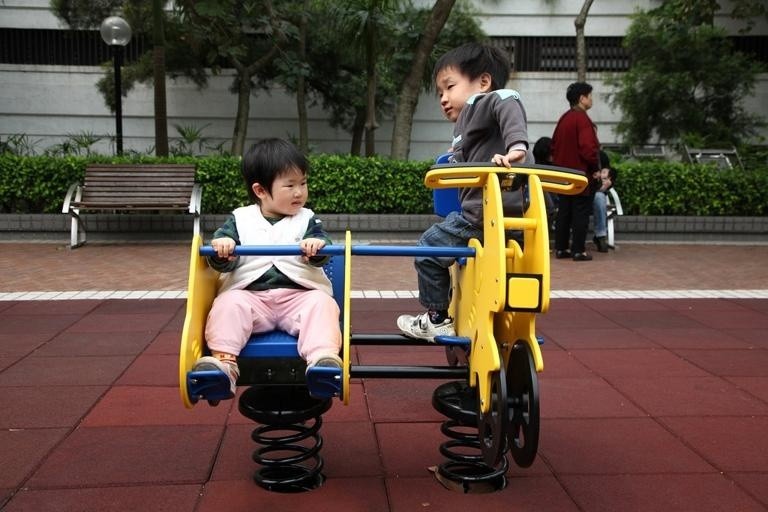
[556, 250, 592, 260]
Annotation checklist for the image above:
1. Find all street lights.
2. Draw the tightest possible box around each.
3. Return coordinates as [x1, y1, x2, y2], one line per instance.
[98, 15, 134, 159]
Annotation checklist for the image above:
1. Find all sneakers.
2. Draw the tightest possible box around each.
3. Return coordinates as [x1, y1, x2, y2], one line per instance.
[594, 236, 608, 252]
[308, 352, 343, 368]
[193, 356, 240, 394]
[396, 312, 456, 344]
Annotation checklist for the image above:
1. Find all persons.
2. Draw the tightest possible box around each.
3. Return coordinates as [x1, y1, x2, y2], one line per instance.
[533, 137, 561, 232]
[550, 82, 602, 261]
[190, 136, 344, 399]
[591, 123, 616, 253]
[398, 43, 537, 345]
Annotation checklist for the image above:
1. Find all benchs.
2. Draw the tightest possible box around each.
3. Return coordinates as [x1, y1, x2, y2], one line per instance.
[62, 163, 204, 250]
